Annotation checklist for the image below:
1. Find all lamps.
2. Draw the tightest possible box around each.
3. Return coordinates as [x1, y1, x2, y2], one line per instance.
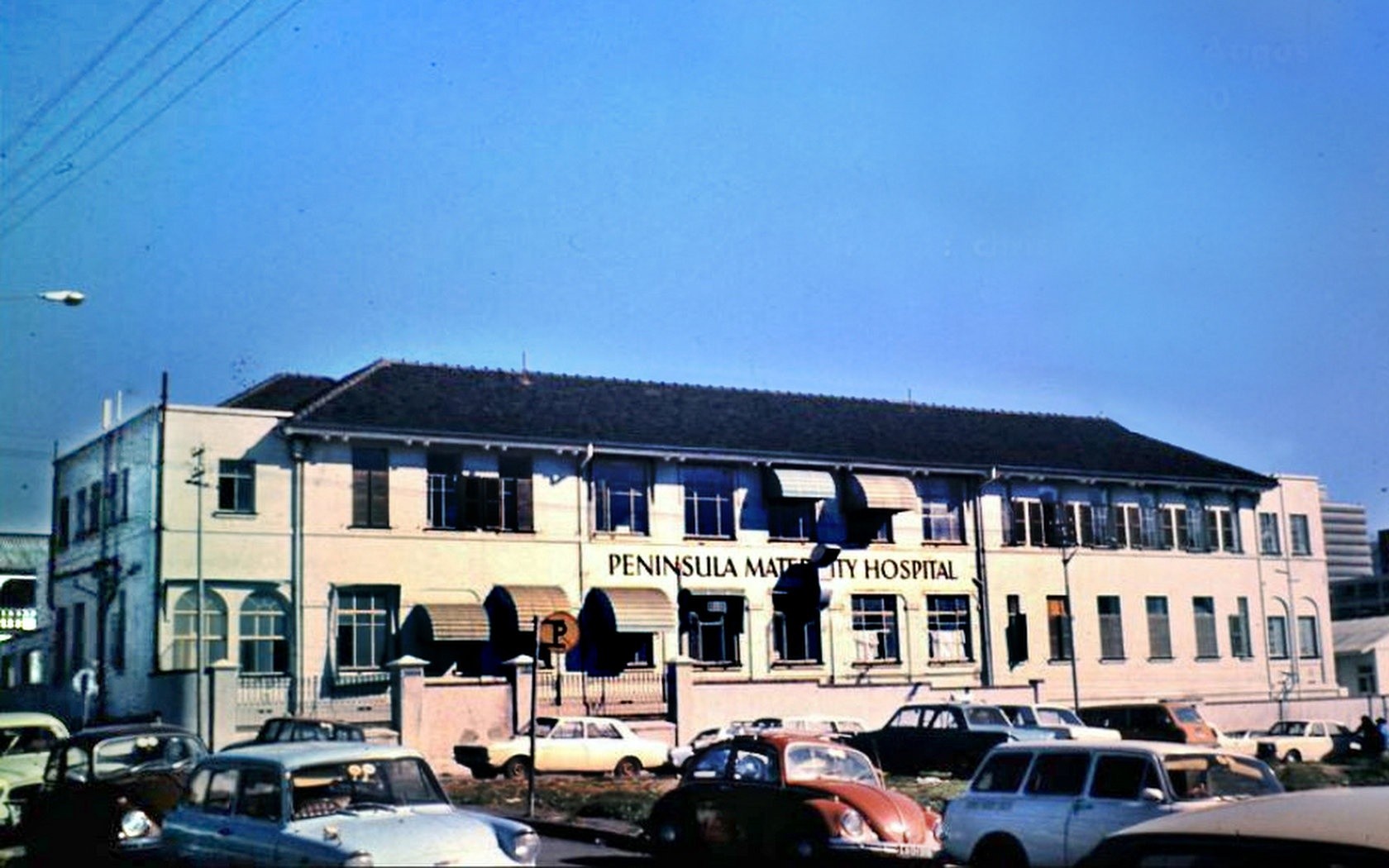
[812, 544, 842, 567]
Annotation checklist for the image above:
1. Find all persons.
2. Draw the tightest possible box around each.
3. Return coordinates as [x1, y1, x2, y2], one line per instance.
[1341, 715, 1389, 759]
[296, 779, 352, 818]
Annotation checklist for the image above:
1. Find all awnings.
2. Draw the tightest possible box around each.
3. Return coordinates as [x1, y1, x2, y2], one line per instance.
[584, 586, 677, 634]
[493, 584, 571, 633]
[415, 604, 491, 643]
[847, 474, 919, 513]
[766, 468, 836, 505]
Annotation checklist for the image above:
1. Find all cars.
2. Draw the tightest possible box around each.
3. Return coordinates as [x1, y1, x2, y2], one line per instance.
[949, 703, 1122, 742]
[1077, 785, 1389, 867]
[43, 724, 219, 867]
[216, 718, 366, 755]
[1069, 701, 1215, 750]
[666, 717, 867, 778]
[163, 744, 546, 867]
[936, 741, 1289, 868]
[0, 713, 88, 830]
[1211, 719, 1366, 769]
[832, 702, 1056, 775]
[644, 734, 945, 868]
[452, 716, 675, 783]
